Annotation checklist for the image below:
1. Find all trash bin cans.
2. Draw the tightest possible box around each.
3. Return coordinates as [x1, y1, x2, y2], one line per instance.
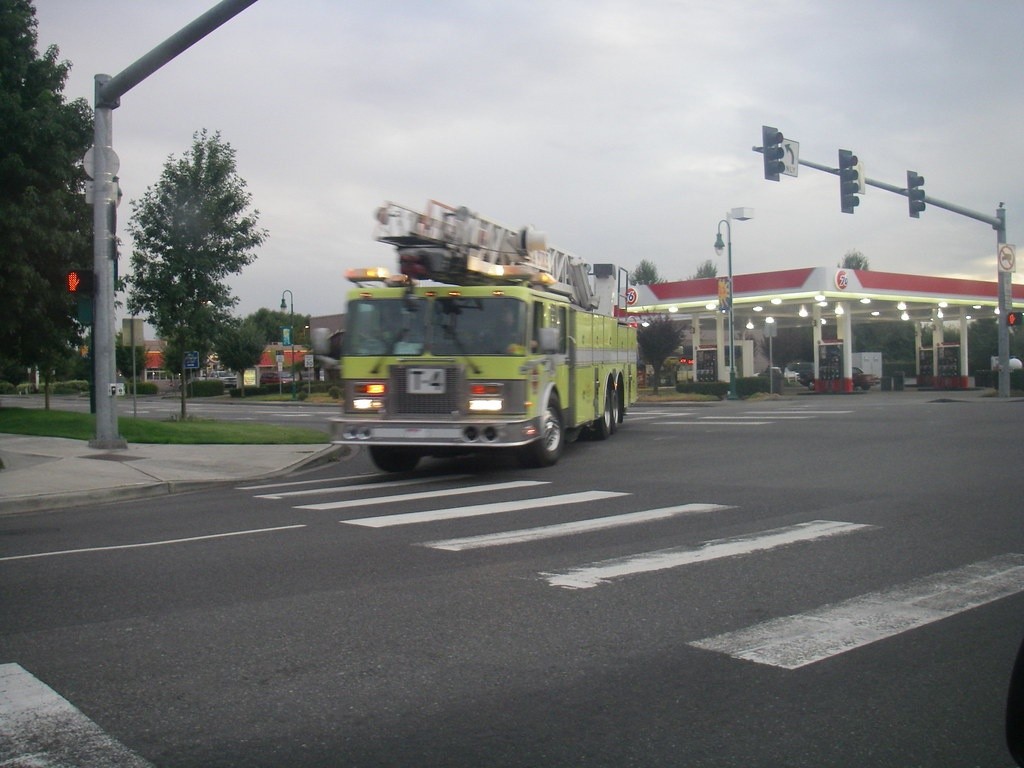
[893, 371, 905, 391]
[881, 376, 892, 391]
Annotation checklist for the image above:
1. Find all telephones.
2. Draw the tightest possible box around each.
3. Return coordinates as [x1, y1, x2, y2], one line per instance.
[108, 382, 125, 396]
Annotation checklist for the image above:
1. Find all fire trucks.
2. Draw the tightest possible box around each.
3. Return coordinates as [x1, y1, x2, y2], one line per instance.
[333, 196, 639, 474]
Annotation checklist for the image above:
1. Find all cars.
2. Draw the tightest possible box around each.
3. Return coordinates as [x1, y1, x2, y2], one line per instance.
[260, 370, 283, 386]
[851, 366, 882, 390]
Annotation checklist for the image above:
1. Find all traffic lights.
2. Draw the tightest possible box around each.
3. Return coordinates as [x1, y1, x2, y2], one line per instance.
[840, 149, 860, 214]
[1007, 311, 1023, 326]
[905, 170, 926, 218]
[67, 269, 93, 293]
[760, 124, 786, 180]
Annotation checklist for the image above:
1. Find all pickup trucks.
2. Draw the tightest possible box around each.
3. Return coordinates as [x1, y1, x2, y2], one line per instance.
[210, 370, 237, 388]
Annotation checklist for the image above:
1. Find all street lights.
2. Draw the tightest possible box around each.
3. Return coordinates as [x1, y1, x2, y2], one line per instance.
[280, 289, 297, 402]
[713, 219, 739, 401]
